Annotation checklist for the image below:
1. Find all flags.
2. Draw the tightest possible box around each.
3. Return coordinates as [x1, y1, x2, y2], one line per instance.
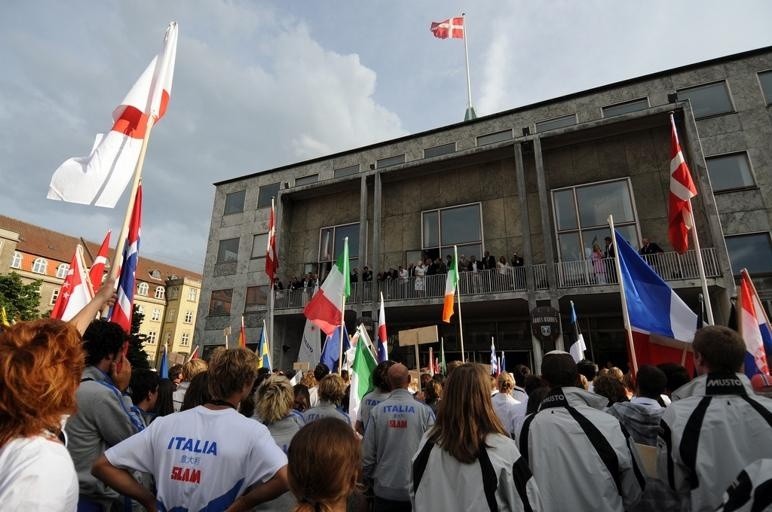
[193, 350, 199, 362]
[380, 296, 388, 362]
[303, 243, 350, 338]
[502, 357, 506, 373]
[159, 349, 170, 379]
[320, 325, 351, 375]
[342, 326, 380, 374]
[443, 251, 457, 323]
[45, 20, 178, 211]
[50, 246, 98, 339]
[627, 331, 696, 390]
[435, 358, 440, 373]
[428, 350, 434, 377]
[740, 273, 772, 393]
[615, 230, 710, 343]
[496, 362, 500, 378]
[692, 299, 706, 329]
[349, 336, 381, 431]
[297, 289, 321, 373]
[89, 232, 111, 297]
[440, 343, 447, 374]
[569, 303, 587, 364]
[431, 17, 464, 39]
[239, 319, 245, 349]
[489, 342, 497, 373]
[104, 184, 142, 372]
[667, 122, 697, 258]
[255, 323, 271, 371]
[264, 204, 279, 291]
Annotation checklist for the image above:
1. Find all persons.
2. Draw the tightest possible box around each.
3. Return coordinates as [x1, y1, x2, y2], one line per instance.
[424, 380, 442, 414]
[605, 365, 667, 510]
[489, 373, 524, 438]
[658, 356, 692, 405]
[251, 373, 305, 512]
[308, 362, 330, 406]
[129, 366, 160, 512]
[286, 417, 361, 511]
[91, 345, 292, 512]
[359, 364, 437, 512]
[487, 374, 499, 395]
[524, 374, 552, 408]
[467, 255, 483, 294]
[603, 237, 618, 283]
[172, 357, 208, 412]
[590, 243, 606, 284]
[274, 272, 321, 309]
[526, 386, 545, 415]
[496, 256, 513, 276]
[179, 370, 210, 411]
[508, 371, 530, 416]
[167, 361, 183, 389]
[575, 355, 636, 407]
[447, 254, 454, 272]
[481, 250, 496, 291]
[407, 364, 544, 512]
[458, 254, 468, 272]
[335, 385, 351, 424]
[239, 367, 271, 417]
[432, 371, 446, 390]
[290, 381, 308, 411]
[637, 237, 664, 266]
[511, 252, 525, 289]
[420, 373, 432, 390]
[351, 256, 446, 291]
[446, 361, 463, 374]
[293, 383, 310, 410]
[342, 368, 351, 385]
[302, 375, 352, 424]
[356, 362, 401, 437]
[252, 373, 272, 403]
[63, 319, 147, 512]
[412, 390, 427, 403]
[655, 325, 772, 512]
[0, 278, 118, 512]
[515, 350, 644, 512]
[145, 378, 174, 423]
